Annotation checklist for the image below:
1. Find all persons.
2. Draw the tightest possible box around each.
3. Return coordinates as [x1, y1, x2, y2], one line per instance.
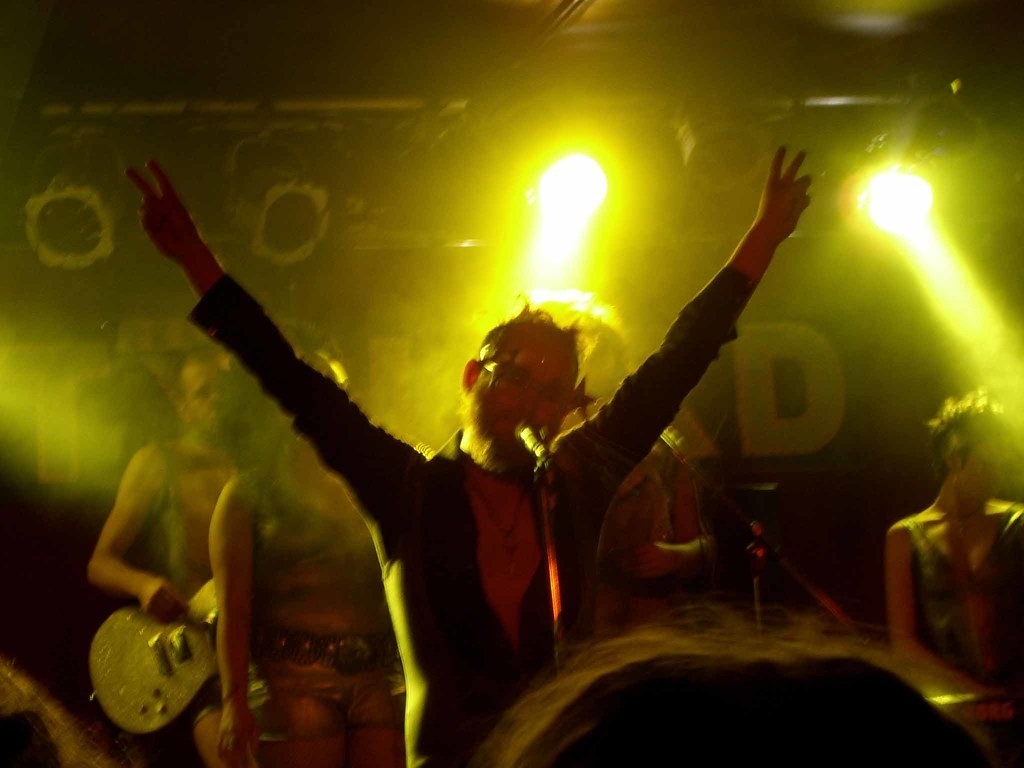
[123, 147, 813, 768]
[87, 344, 396, 768]
[468, 623, 995, 768]
[886, 395, 1024, 697]
[594, 462, 716, 640]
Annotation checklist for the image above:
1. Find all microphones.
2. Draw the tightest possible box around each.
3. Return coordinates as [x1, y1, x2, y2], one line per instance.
[659, 430, 682, 452]
[516, 418, 550, 462]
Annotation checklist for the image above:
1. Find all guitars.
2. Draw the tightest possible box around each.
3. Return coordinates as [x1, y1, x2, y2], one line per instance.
[88, 562, 235, 735]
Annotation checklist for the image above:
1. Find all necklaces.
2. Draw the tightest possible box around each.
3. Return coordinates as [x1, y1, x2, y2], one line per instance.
[469, 476, 527, 561]
[947, 507, 980, 536]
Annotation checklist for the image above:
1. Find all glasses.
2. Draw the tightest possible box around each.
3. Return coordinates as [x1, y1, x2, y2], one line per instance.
[478, 356, 573, 414]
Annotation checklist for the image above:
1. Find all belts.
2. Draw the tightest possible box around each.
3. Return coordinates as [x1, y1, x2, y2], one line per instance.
[249, 628, 398, 677]
